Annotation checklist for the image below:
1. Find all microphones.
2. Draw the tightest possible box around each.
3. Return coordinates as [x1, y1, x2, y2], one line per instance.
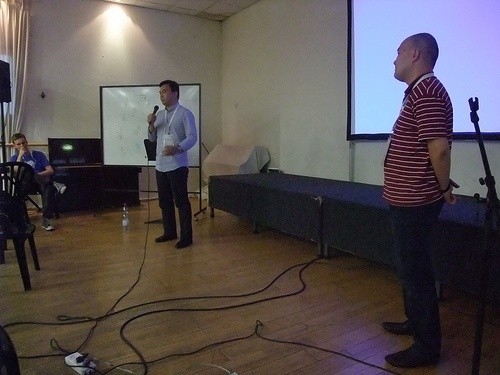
[149, 105, 159, 124]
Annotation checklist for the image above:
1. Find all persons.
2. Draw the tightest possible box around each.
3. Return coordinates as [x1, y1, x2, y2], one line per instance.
[8, 133, 67, 231]
[381, 32, 461, 368]
[147, 80, 197, 248]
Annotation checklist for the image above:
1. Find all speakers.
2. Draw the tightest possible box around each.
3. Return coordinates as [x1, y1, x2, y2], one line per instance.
[0, 60, 11, 103]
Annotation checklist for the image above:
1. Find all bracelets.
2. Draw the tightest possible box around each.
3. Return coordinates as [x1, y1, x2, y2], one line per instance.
[438, 184, 450, 193]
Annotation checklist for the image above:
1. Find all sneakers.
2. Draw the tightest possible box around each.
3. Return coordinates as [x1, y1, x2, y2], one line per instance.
[41, 217, 55, 231]
[54, 181, 67, 195]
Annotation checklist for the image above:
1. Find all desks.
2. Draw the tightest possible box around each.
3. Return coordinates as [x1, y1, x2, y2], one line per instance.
[42, 166, 142, 216]
[208, 174, 500, 306]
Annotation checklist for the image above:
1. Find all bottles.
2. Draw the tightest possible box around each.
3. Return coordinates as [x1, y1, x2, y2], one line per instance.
[122, 202, 130, 233]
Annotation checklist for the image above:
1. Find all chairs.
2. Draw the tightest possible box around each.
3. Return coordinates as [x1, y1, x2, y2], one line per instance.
[0, 161, 59, 291]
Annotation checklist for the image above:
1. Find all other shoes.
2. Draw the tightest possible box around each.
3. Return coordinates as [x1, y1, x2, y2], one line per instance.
[155, 233, 177, 243]
[174, 239, 193, 249]
[382, 320, 412, 336]
[385, 343, 440, 366]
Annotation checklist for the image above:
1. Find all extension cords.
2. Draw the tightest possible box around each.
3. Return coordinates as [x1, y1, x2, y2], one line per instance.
[65, 352, 96, 375]
